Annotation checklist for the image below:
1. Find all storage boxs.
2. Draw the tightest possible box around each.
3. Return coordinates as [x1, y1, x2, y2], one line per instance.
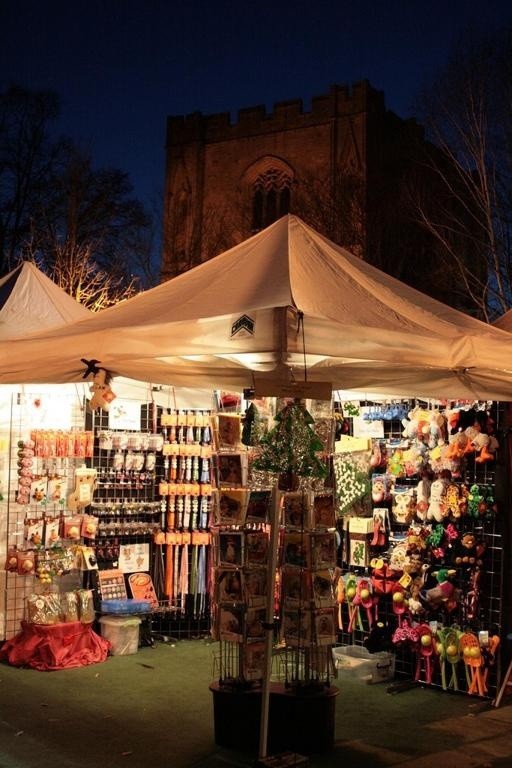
[332, 646, 396, 684]
[98, 615, 143, 653]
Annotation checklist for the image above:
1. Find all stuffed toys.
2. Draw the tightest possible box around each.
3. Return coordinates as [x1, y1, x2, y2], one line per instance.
[330, 402, 499, 695]
[89, 368, 115, 413]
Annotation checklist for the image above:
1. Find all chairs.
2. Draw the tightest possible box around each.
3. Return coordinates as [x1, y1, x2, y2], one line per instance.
[129, 572, 182, 650]
